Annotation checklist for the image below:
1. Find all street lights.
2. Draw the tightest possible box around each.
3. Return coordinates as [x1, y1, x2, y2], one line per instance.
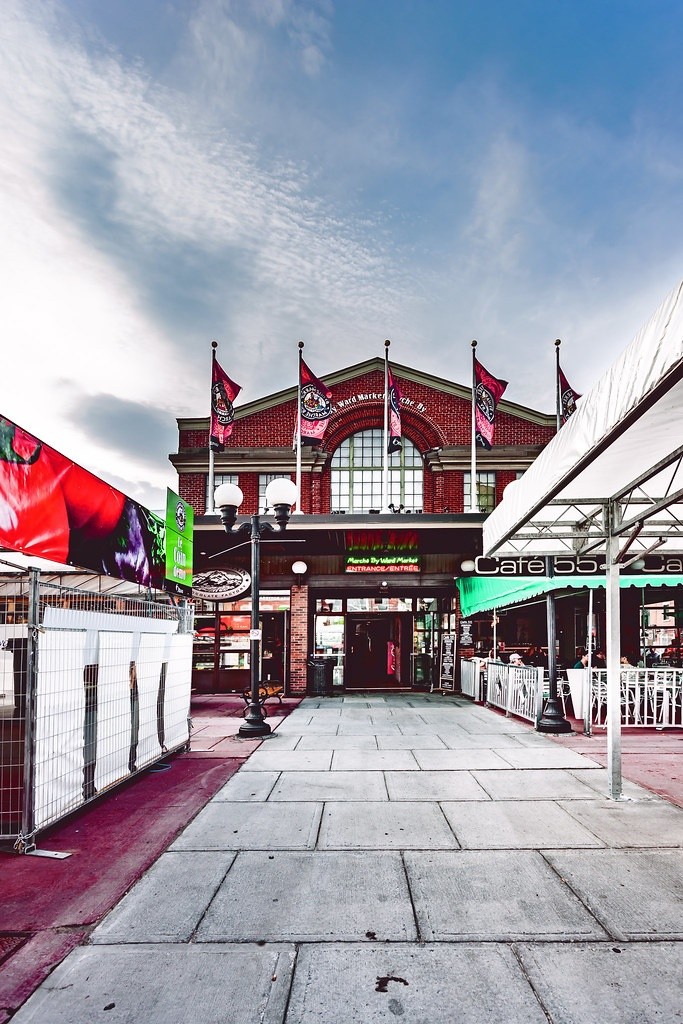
[213, 478, 301, 736]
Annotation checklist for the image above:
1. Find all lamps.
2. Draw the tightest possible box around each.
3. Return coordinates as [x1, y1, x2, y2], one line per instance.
[461, 560, 475, 578]
[291, 562, 308, 585]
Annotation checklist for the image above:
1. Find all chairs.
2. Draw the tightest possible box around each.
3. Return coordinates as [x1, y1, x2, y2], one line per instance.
[542, 668, 570, 720]
[590, 672, 683, 729]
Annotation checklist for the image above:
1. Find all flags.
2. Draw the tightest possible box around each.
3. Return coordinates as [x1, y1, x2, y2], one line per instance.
[210, 358, 242, 453]
[559, 366, 581, 424]
[292, 358, 332, 454]
[474, 357, 509, 451]
[388, 368, 402, 454]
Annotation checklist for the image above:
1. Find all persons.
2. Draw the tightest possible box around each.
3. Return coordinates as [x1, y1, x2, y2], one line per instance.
[572, 647, 660, 710]
[468, 643, 547, 703]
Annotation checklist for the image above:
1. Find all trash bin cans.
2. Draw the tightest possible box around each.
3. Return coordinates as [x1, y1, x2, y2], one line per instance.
[310, 658, 336, 693]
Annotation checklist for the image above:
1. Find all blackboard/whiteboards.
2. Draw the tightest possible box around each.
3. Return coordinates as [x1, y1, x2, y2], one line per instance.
[439, 634, 457, 691]
